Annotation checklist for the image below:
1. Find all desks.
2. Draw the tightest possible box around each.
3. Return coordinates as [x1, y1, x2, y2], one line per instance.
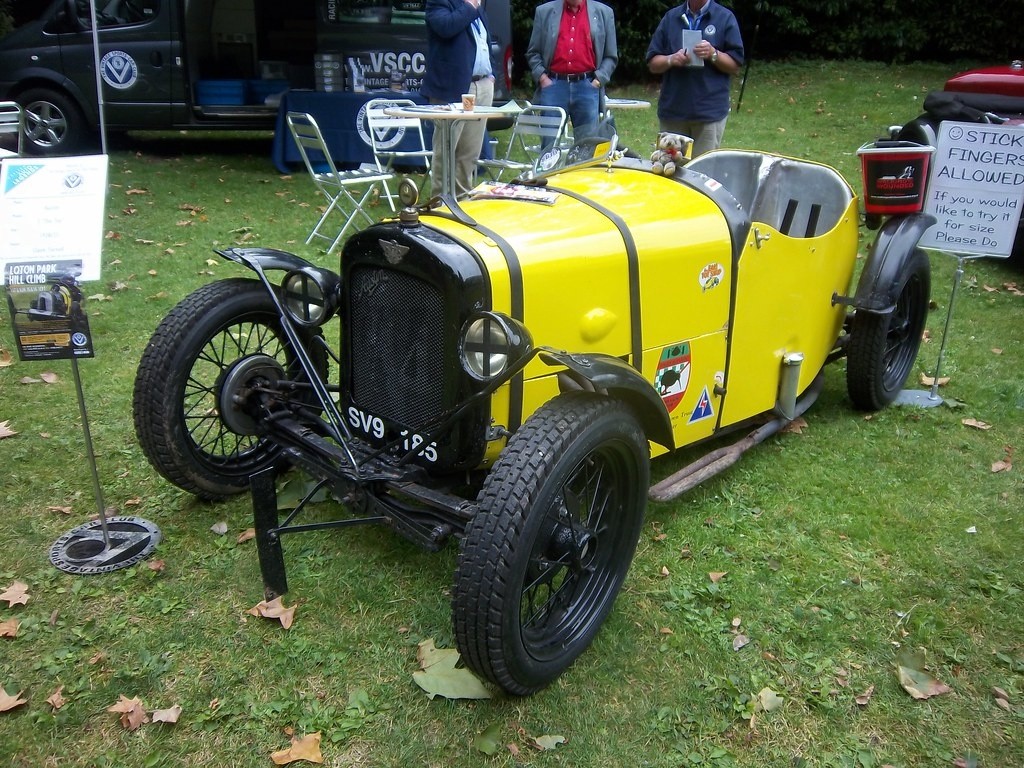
[606, 98, 652, 117]
[272, 89, 491, 178]
[383, 105, 524, 200]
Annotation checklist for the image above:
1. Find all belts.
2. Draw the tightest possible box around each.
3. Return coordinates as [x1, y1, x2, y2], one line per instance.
[471, 75, 486, 82]
[548, 71, 595, 82]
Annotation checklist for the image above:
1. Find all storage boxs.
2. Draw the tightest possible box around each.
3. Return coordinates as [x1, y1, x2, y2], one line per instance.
[249, 78, 285, 95]
[195, 79, 250, 106]
[488, 137, 499, 159]
[312, 54, 345, 92]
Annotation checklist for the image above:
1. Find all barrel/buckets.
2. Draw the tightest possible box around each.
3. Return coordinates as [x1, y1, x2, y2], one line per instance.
[313, 50, 343, 92]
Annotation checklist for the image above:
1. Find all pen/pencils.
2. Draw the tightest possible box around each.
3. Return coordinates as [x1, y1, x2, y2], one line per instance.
[684, 48, 688, 56]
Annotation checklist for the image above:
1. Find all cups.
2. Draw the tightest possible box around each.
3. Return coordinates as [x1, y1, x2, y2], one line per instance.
[461, 94, 475, 111]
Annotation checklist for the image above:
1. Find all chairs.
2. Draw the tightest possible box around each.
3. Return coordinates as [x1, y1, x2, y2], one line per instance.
[366, 98, 434, 210]
[510, 102, 575, 168]
[471, 102, 571, 185]
[285, 110, 396, 254]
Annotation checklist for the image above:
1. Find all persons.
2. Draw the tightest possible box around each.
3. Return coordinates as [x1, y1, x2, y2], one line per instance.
[422, 0, 496, 205]
[646, 0, 744, 158]
[525, 0, 618, 169]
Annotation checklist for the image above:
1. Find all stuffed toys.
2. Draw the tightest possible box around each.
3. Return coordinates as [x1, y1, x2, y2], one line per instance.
[650, 135, 683, 176]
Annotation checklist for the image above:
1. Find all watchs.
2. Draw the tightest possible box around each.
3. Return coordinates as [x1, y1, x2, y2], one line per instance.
[711, 49, 718, 62]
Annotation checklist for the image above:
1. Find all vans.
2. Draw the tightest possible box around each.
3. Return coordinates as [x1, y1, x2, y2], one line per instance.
[1, 2, 522, 159]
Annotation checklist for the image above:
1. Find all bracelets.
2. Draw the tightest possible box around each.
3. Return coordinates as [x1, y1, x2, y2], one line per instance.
[468, 1, 476, 9]
[540, 75, 547, 83]
[668, 55, 672, 65]
[593, 78, 600, 87]
